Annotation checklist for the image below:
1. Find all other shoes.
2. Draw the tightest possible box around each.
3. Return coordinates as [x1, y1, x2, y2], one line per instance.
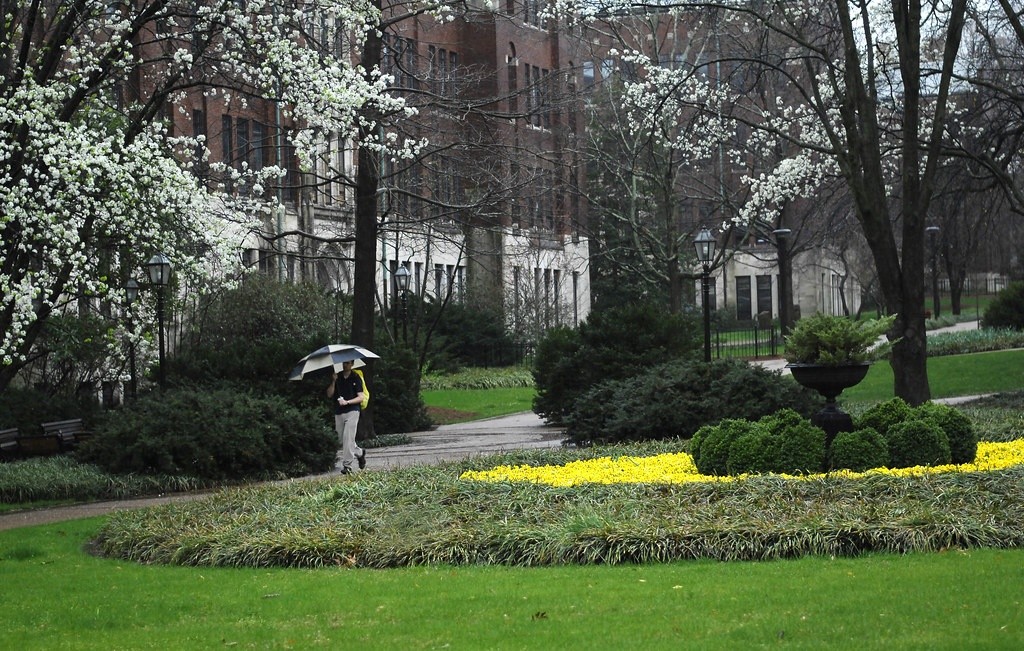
[357, 449, 366, 469]
[341, 467, 352, 474]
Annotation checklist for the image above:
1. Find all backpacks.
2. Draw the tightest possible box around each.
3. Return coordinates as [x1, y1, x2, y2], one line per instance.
[352, 370, 370, 410]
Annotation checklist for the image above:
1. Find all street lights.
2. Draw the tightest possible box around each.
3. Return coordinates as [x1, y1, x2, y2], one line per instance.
[144, 247, 173, 394]
[394, 264, 413, 349]
[692, 221, 717, 363]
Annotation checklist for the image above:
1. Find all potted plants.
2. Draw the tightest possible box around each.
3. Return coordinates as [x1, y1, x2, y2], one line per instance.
[783, 310, 904, 415]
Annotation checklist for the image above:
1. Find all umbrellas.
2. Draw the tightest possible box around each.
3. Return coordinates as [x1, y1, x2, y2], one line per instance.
[289, 344, 380, 400]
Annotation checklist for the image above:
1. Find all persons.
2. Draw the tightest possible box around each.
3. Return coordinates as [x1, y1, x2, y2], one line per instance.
[327, 359, 367, 473]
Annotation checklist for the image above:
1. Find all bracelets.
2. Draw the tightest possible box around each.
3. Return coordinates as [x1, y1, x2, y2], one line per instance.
[347, 400, 349, 405]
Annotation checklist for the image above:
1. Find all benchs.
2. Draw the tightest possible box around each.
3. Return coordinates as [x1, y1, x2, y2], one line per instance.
[14, 434, 66, 460]
[72, 432, 91, 440]
[39, 418, 90, 442]
[0, 426, 26, 458]
